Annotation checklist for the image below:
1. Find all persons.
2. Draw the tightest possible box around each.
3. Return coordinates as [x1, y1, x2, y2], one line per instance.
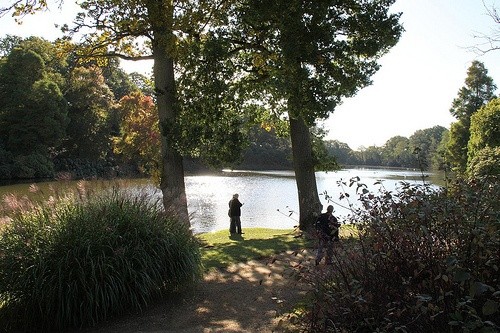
[312, 205, 339, 268]
[228, 193, 246, 235]
